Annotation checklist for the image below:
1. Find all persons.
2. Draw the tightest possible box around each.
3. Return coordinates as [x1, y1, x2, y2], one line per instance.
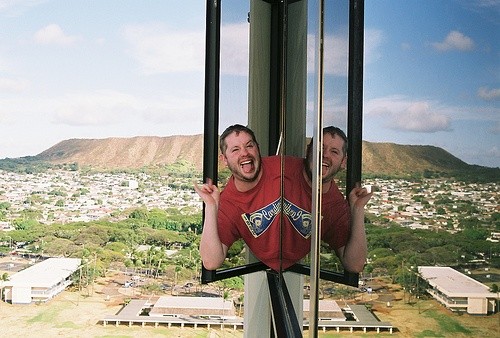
[282, 126, 375, 274]
[192, 124, 282, 271]
